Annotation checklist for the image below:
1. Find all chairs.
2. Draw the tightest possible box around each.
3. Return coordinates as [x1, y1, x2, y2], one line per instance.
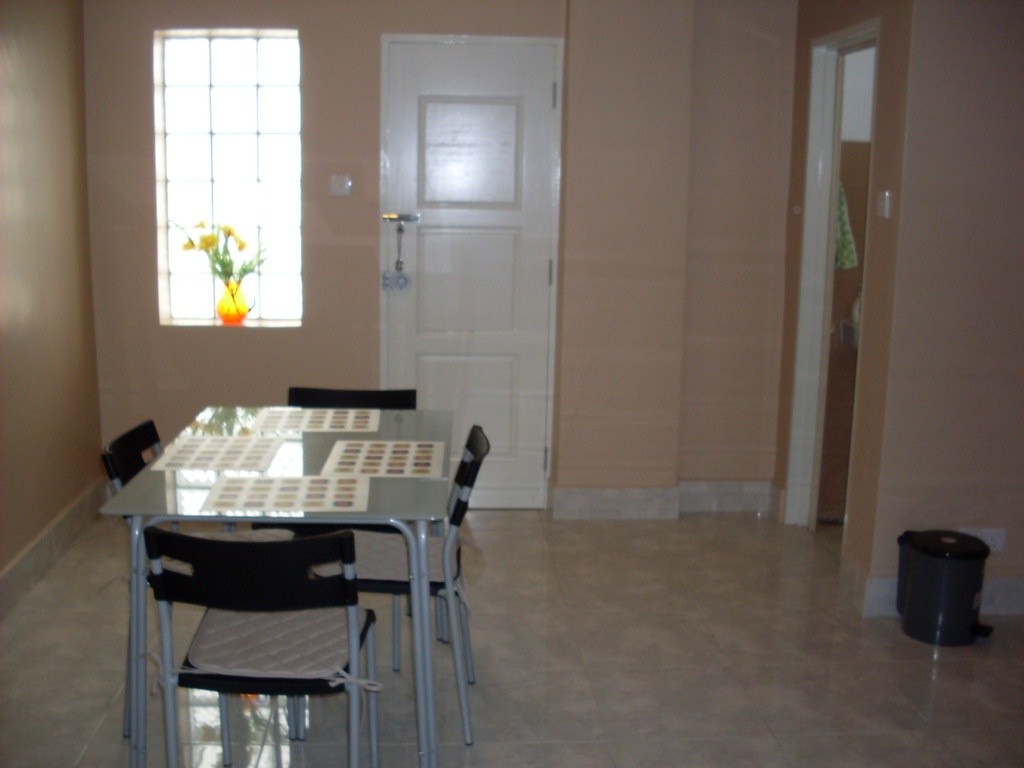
[101, 387, 491, 768]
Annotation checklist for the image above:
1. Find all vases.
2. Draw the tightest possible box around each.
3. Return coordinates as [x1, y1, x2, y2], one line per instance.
[215, 282, 248, 326]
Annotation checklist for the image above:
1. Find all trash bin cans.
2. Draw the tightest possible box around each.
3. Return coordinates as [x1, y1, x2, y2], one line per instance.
[895, 529, 990, 647]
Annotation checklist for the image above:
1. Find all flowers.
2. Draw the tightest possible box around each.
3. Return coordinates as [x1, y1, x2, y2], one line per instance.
[182, 222, 267, 317]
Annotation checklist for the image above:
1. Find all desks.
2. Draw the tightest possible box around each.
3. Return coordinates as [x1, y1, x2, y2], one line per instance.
[99, 405, 455, 768]
